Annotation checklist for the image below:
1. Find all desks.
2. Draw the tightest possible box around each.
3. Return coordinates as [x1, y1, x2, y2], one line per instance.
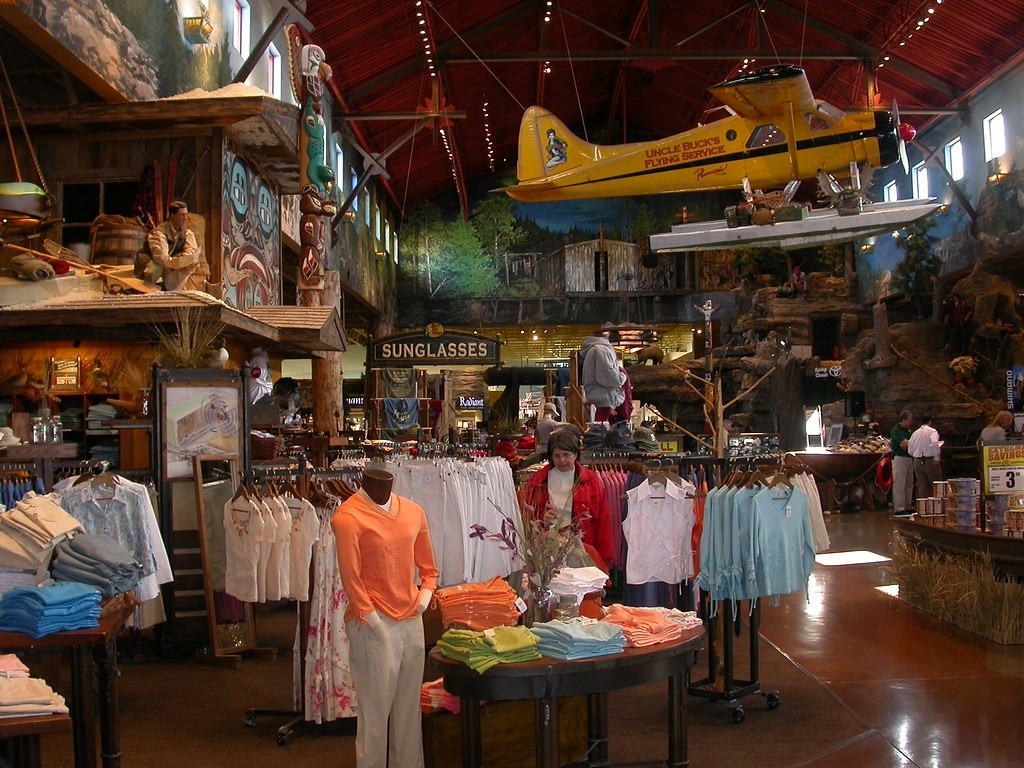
[429, 625, 707, 767]
[1, 604, 135, 768]
[0, 713, 71, 768]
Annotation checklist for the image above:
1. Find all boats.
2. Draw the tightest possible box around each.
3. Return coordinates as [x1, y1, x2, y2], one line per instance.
[650, 193, 945, 252]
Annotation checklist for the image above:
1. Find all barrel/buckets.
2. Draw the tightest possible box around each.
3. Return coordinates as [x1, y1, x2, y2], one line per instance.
[0, 232, 46, 277]
[93, 225, 146, 265]
[68, 242, 91, 263]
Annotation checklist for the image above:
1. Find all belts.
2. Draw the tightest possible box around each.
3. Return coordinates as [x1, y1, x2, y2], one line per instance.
[917, 456, 935, 459]
[895, 454, 913, 458]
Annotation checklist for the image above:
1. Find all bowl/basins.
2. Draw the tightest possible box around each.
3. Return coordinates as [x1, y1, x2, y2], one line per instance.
[945, 477, 1008, 532]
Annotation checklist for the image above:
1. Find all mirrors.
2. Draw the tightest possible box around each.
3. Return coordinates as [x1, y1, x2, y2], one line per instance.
[194, 454, 276, 658]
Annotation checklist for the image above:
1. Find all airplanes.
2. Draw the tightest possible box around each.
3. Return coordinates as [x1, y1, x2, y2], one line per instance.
[488, 63, 917, 204]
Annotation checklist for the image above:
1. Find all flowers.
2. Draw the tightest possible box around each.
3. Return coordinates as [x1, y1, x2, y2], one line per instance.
[469, 496, 592, 586]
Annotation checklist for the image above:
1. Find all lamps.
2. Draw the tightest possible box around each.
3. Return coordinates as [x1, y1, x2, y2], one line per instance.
[186, 15, 214, 44]
[988, 172, 1008, 185]
[376, 251, 386, 262]
[861, 244, 874, 254]
[935, 205, 951, 217]
[343, 211, 356, 222]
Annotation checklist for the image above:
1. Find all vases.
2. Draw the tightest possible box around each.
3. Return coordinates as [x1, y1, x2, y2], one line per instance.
[525, 588, 550, 626]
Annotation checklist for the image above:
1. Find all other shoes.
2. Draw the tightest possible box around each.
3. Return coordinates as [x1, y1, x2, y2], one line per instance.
[894, 510, 917, 515]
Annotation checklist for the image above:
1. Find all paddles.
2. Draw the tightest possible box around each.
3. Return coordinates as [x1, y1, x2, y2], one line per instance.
[0, 237, 162, 294]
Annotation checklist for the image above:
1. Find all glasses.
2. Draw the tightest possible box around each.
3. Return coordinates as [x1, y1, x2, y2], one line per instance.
[552, 451, 574, 460]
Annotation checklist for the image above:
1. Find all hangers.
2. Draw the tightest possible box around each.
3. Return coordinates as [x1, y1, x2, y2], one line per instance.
[590, 452, 705, 499]
[200, 471, 231, 487]
[374, 438, 507, 464]
[59, 461, 145, 493]
[716, 456, 811, 500]
[1, 461, 44, 490]
[232, 469, 303, 513]
[311, 467, 365, 508]
[335, 448, 368, 463]
[278, 450, 309, 468]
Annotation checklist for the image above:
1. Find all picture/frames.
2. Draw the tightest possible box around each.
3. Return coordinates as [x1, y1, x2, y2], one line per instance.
[162, 380, 246, 481]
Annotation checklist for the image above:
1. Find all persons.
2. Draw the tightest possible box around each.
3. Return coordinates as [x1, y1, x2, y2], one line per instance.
[908, 413, 943, 512]
[976, 411, 1013, 456]
[714, 420, 732, 459]
[331, 468, 438, 768]
[580, 331, 628, 409]
[890, 410, 918, 518]
[134, 201, 200, 292]
[536, 403, 570, 455]
[518, 428, 616, 571]
[941, 292, 974, 356]
[558, 524, 612, 620]
[791, 266, 805, 289]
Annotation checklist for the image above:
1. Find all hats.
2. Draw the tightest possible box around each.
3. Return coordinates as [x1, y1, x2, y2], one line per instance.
[544, 403, 560, 416]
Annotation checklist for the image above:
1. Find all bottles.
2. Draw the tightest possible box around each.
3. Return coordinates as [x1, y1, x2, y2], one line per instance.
[28, 415, 63, 444]
[552, 591, 604, 620]
